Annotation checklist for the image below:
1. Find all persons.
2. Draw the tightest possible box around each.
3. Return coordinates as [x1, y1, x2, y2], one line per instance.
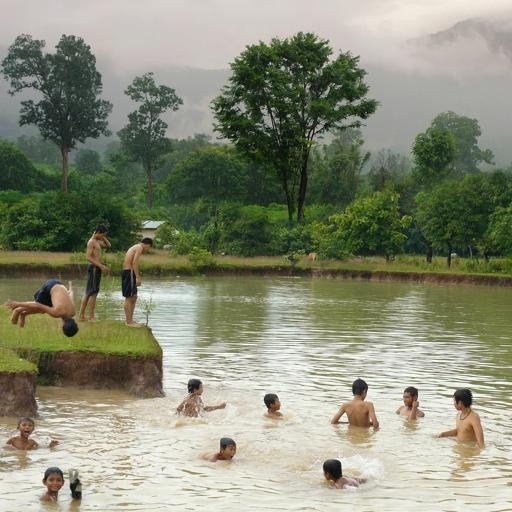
[7, 418, 59, 450]
[263, 394, 284, 420]
[439, 389, 485, 449]
[78, 225, 111, 322]
[175, 378, 227, 417]
[323, 459, 366, 489]
[39, 467, 65, 503]
[396, 387, 424, 421]
[198, 437, 236, 462]
[121, 238, 154, 328]
[5, 278, 79, 337]
[331, 377, 380, 429]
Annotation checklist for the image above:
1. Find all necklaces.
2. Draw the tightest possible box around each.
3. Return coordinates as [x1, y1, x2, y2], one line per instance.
[460, 408, 472, 421]
